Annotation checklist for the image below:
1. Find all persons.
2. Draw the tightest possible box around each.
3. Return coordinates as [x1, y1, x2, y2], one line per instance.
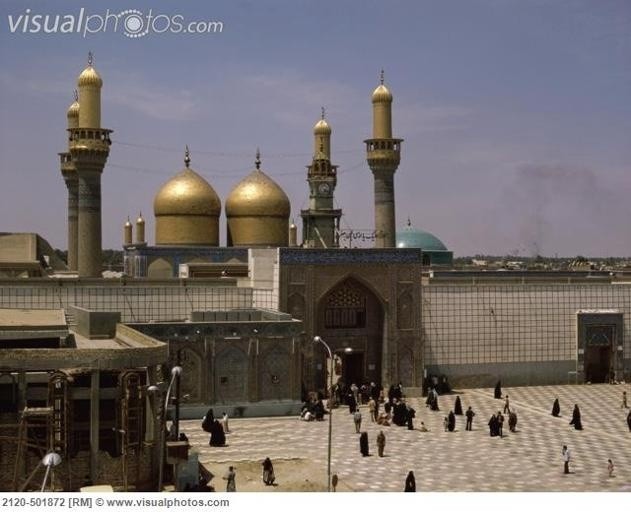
[622, 391, 630, 408]
[210, 420, 225, 446]
[301, 374, 519, 438]
[553, 399, 560, 416]
[610, 366, 617, 384]
[220, 411, 230, 434]
[377, 430, 386, 456]
[361, 432, 370, 456]
[405, 471, 416, 492]
[608, 459, 614, 477]
[262, 456, 276, 485]
[203, 408, 214, 432]
[562, 445, 570, 474]
[223, 465, 236, 492]
[573, 409, 583, 430]
[570, 403, 579, 425]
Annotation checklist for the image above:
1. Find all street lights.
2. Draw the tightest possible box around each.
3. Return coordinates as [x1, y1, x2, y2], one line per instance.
[148, 366, 183, 491]
[38, 452, 62, 491]
[313, 336, 354, 492]
